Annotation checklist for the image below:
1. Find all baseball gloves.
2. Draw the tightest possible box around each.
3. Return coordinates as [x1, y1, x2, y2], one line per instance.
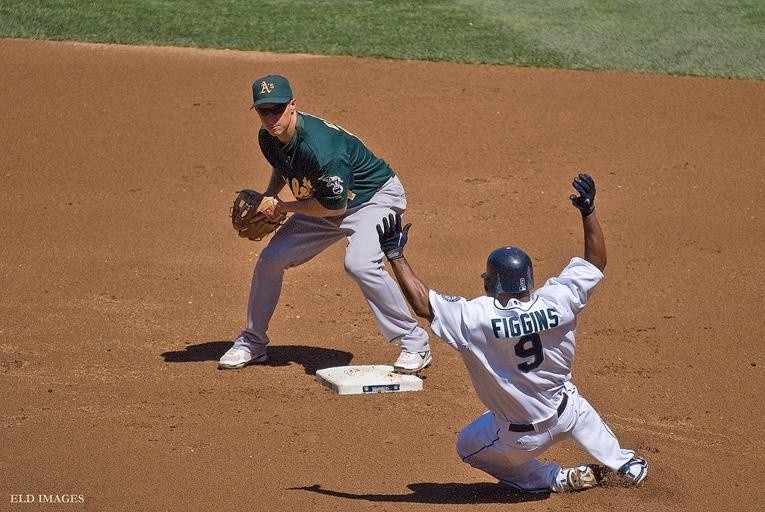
[230, 189, 286, 242]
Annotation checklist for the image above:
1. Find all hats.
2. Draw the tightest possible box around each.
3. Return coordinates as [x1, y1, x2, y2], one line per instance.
[249, 73, 293, 111]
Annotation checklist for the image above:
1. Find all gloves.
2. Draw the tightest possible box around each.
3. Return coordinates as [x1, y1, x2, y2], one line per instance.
[568, 172, 599, 218]
[375, 213, 412, 262]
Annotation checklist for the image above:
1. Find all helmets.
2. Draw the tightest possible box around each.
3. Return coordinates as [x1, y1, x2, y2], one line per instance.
[481, 245, 535, 298]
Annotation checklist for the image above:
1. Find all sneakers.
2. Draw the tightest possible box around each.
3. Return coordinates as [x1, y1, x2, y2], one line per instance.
[616, 454, 649, 486]
[552, 463, 610, 492]
[218, 330, 269, 371]
[393, 343, 433, 374]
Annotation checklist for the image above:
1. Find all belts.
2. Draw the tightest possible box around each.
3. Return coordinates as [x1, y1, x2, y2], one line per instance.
[494, 391, 569, 432]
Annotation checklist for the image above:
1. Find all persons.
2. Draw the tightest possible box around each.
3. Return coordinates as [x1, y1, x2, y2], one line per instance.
[218, 75, 433, 375]
[376, 172, 650, 494]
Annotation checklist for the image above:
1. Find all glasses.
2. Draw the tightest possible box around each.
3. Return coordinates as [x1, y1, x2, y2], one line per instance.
[255, 99, 291, 117]
